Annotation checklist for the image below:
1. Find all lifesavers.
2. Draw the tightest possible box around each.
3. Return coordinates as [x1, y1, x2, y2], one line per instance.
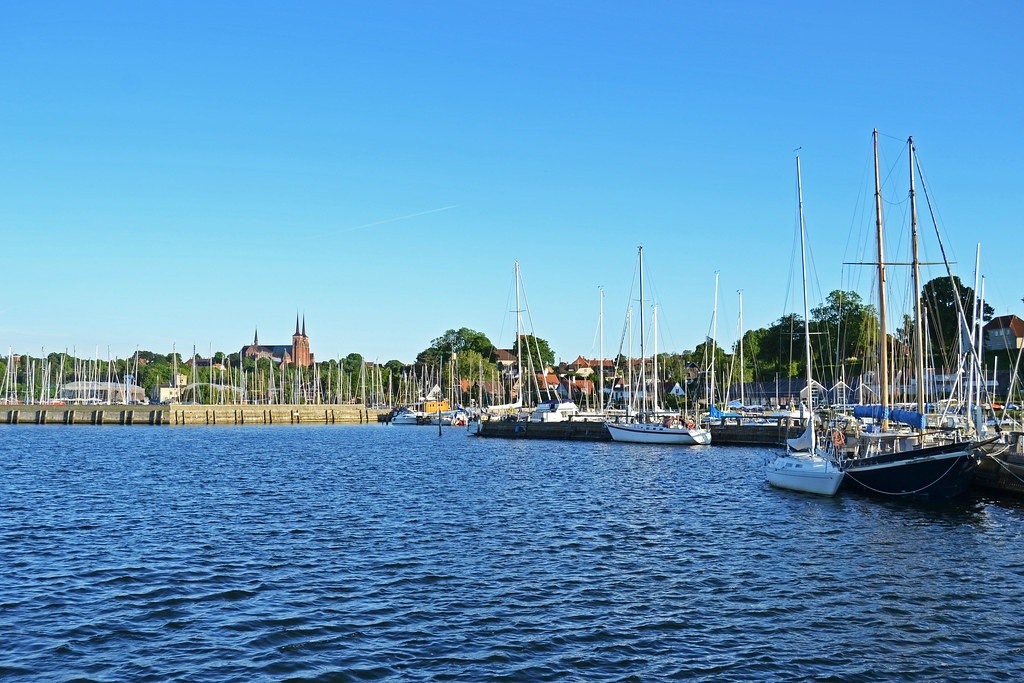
[832, 430, 846, 446]
[687, 421, 695, 429]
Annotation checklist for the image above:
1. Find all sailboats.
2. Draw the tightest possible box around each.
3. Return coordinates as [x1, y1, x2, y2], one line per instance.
[392, 126, 1024, 517]
